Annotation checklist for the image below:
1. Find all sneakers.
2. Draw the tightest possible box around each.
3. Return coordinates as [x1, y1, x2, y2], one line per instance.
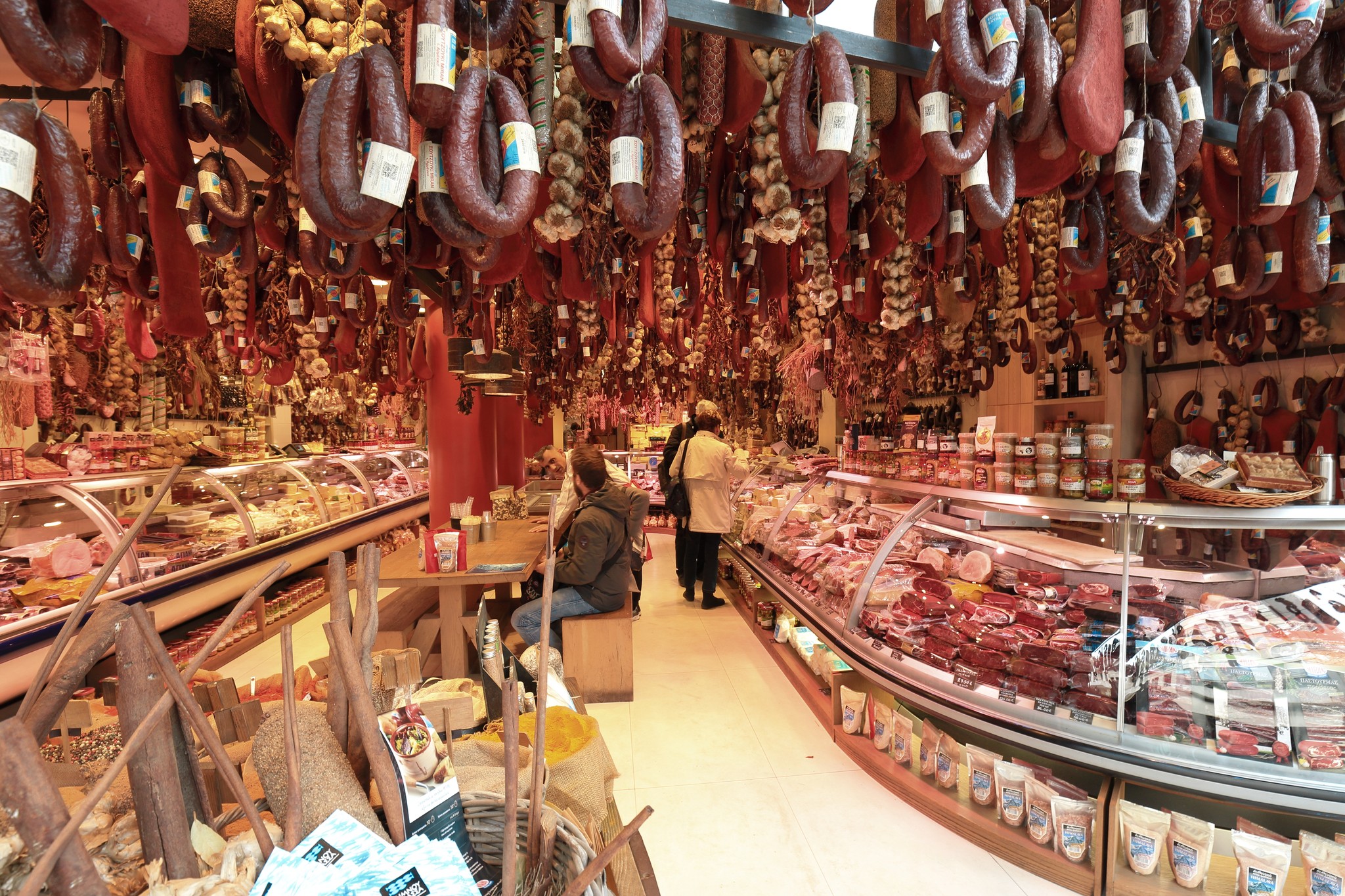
[632, 607, 640, 621]
[635, 605, 641, 615]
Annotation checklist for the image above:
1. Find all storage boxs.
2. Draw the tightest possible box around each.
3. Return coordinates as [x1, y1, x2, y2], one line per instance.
[165, 510, 213, 525]
[165, 521, 211, 533]
[1162, 444, 1313, 491]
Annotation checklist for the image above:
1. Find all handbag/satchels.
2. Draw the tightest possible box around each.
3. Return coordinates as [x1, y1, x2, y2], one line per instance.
[520, 570, 567, 604]
[658, 458, 669, 492]
[630, 549, 646, 571]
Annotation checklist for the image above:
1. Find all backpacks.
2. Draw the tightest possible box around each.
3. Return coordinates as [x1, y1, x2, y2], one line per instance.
[664, 438, 691, 518]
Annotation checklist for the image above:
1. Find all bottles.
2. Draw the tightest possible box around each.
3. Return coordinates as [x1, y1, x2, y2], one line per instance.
[1037, 350, 1099, 400]
[1067, 411, 1074, 426]
[482, 619, 504, 689]
[518, 681, 538, 713]
[228, 422, 236, 428]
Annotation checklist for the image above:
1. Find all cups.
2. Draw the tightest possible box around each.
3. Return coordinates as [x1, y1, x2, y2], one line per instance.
[1308, 454, 1336, 506]
[480, 510, 498, 542]
[390, 723, 438, 781]
[449, 496, 474, 531]
[460, 524, 481, 544]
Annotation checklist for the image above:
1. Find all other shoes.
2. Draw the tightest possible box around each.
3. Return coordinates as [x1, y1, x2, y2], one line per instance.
[678, 576, 685, 587]
[696, 576, 704, 581]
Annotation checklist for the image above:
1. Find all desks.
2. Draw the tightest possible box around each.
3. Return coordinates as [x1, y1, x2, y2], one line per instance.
[347, 515, 548, 677]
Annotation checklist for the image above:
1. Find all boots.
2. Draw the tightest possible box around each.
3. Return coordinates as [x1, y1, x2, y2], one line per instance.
[683, 585, 695, 602]
[701, 589, 725, 610]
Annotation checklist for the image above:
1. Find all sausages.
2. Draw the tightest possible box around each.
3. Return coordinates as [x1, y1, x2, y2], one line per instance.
[296, 0, 1345, 447]
[1151, 407, 1345, 572]
[0, 0, 302, 426]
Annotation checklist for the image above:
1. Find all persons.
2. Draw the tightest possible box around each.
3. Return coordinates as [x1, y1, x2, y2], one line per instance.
[669, 409, 750, 610]
[511, 445, 631, 654]
[534, 445, 653, 621]
[564, 422, 580, 451]
[663, 400, 724, 588]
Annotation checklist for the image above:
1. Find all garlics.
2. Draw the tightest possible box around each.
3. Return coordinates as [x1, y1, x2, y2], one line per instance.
[27, 0, 1328, 480]
[0, 790, 284, 896]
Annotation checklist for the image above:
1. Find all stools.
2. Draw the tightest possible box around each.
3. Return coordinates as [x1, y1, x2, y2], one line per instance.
[562, 591, 633, 704]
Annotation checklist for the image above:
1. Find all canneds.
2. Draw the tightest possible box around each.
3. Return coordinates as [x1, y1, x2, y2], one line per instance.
[390, 725, 438, 782]
[344, 559, 357, 578]
[843, 427, 1146, 500]
[72, 575, 325, 704]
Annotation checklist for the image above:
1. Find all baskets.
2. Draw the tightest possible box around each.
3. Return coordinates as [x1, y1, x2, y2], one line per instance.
[1151, 464, 1328, 508]
